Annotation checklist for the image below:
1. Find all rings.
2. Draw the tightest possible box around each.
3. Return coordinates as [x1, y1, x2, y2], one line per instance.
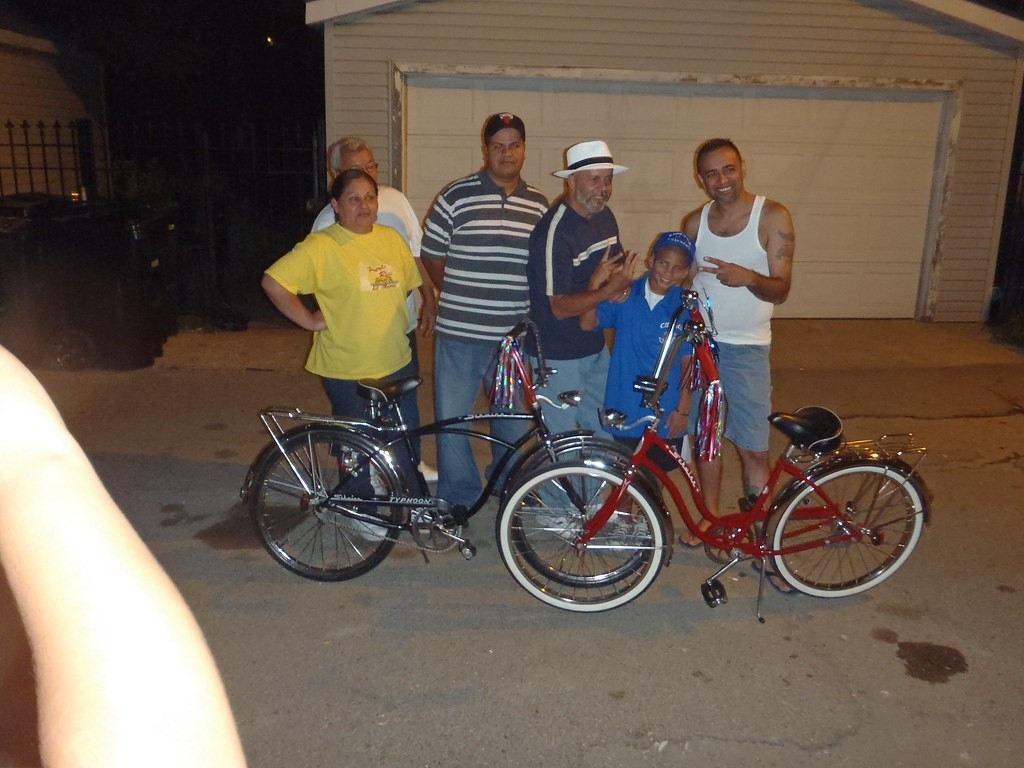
[623, 292, 627, 295]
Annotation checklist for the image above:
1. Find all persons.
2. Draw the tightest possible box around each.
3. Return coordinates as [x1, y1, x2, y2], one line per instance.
[419, 113, 543, 515]
[678, 138, 802, 598]
[260, 167, 444, 541]
[311, 137, 442, 493]
[0, 339, 246, 768]
[578, 231, 696, 532]
[527, 141, 625, 541]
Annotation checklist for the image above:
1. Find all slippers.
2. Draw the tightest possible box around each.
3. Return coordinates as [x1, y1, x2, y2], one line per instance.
[680, 535, 705, 548]
[751, 559, 798, 595]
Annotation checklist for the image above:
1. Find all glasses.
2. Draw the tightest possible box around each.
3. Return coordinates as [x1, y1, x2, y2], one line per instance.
[335, 160, 378, 174]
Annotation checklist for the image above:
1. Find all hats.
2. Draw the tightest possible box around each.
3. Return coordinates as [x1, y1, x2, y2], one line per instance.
[552, 140, 629, 179]
[652, 231, 696, 269]
[484, 112, 526, 141]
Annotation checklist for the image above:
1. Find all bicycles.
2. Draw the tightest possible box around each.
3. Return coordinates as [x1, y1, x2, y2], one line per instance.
[241, 321, 661, 587]
[496, 289, 933, 624]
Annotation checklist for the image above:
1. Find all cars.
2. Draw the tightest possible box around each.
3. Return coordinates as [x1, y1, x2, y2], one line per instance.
[0, 192, 179, 371]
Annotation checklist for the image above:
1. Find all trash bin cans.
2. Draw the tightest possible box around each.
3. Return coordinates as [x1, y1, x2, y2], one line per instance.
[39, 198, 163, 371]
[0, 191, 74, 363]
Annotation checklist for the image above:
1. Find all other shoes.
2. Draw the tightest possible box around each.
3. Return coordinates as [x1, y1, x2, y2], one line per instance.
[416, 459, 440, 484]
[370, 462, 389, 498]
[410, 510, 440, 534]
[536, 513, 582, 540]
[351, 512, 388, 543]
[489, 490, 542, 507]
[437, 515, 468, 530]
[633, 515, 649, 552]
[609, 520, 634, 550]
[570, 503, 618, 523]
